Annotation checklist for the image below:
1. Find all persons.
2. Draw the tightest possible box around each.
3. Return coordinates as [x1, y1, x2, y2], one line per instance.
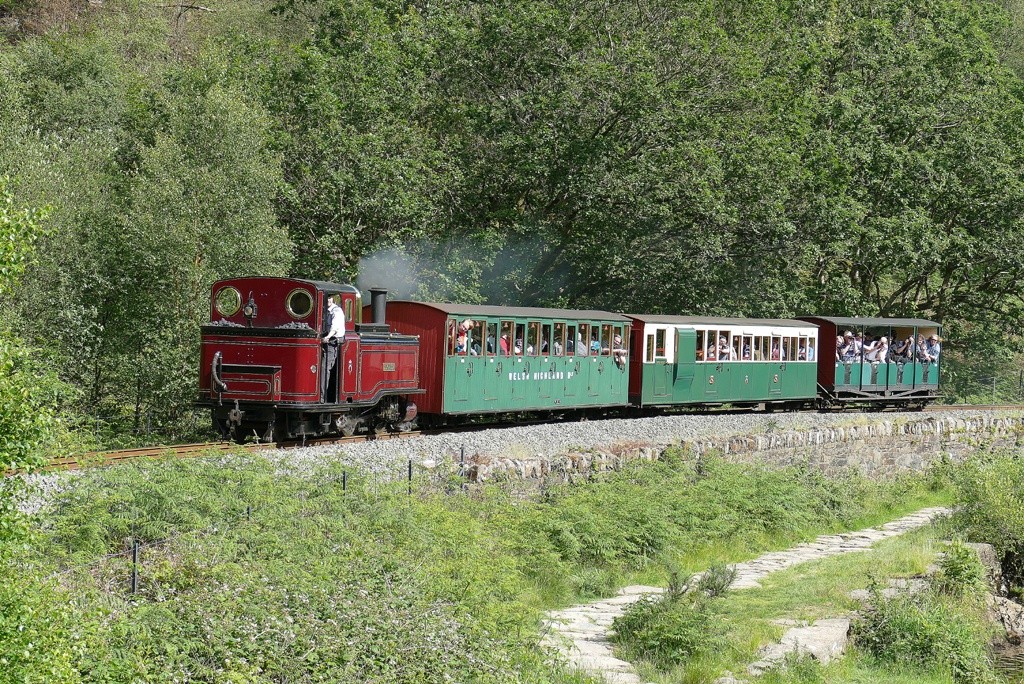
[448, 320, 628, 365]
[836, 329, 941, 365]
[699, 334, 813, 361]
[320, 294, 346, 404]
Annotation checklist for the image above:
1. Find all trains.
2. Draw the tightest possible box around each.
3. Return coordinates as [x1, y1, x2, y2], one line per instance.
[192, 275, 943, 446]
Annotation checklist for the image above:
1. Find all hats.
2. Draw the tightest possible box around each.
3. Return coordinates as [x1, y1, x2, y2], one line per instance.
[930, 334, 938, 340]
